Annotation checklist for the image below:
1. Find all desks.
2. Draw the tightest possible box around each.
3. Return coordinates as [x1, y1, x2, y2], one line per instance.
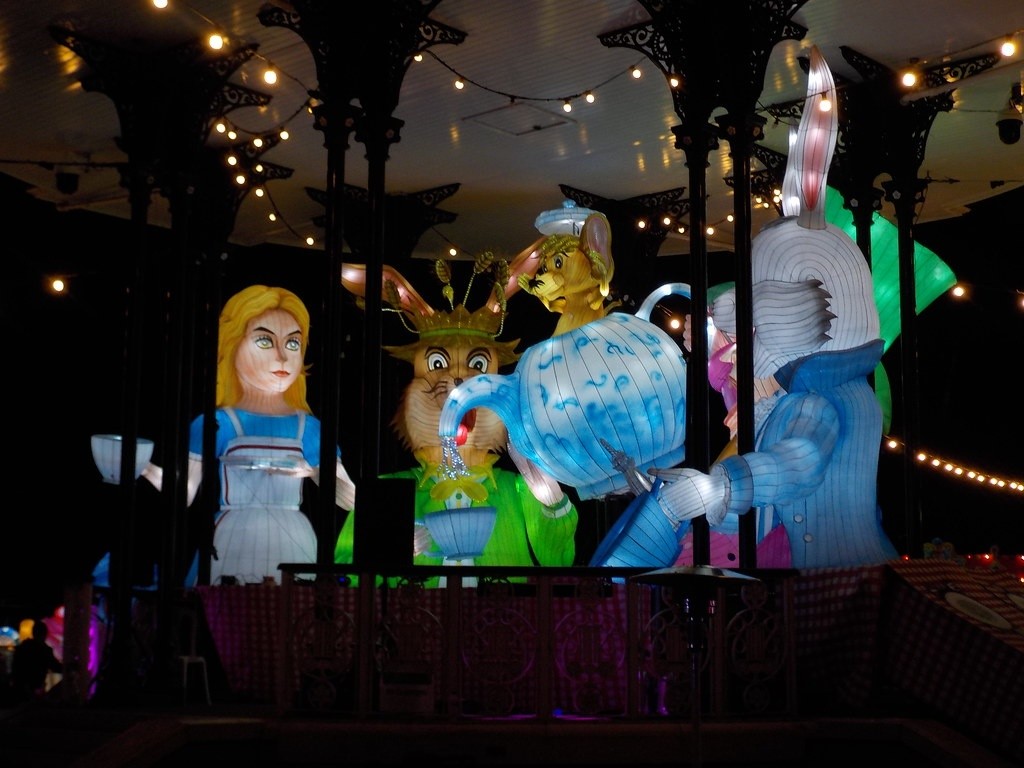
[196, 583, 691, 718]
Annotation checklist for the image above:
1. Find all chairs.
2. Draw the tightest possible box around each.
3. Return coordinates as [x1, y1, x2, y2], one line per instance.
[634, 581, 707, 715]
[547, 574, 630, 726]
[286, 582, 362, 717]
[377, 579, 449, 715]
[718, 578, 793, 718]
[170, 598, 213, 710]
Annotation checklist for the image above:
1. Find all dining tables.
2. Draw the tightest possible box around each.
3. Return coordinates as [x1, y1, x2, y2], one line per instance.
[451, 578, 552, 714]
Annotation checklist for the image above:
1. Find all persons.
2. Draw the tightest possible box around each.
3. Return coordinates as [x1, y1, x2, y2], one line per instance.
[12, 620, 81, 700]
